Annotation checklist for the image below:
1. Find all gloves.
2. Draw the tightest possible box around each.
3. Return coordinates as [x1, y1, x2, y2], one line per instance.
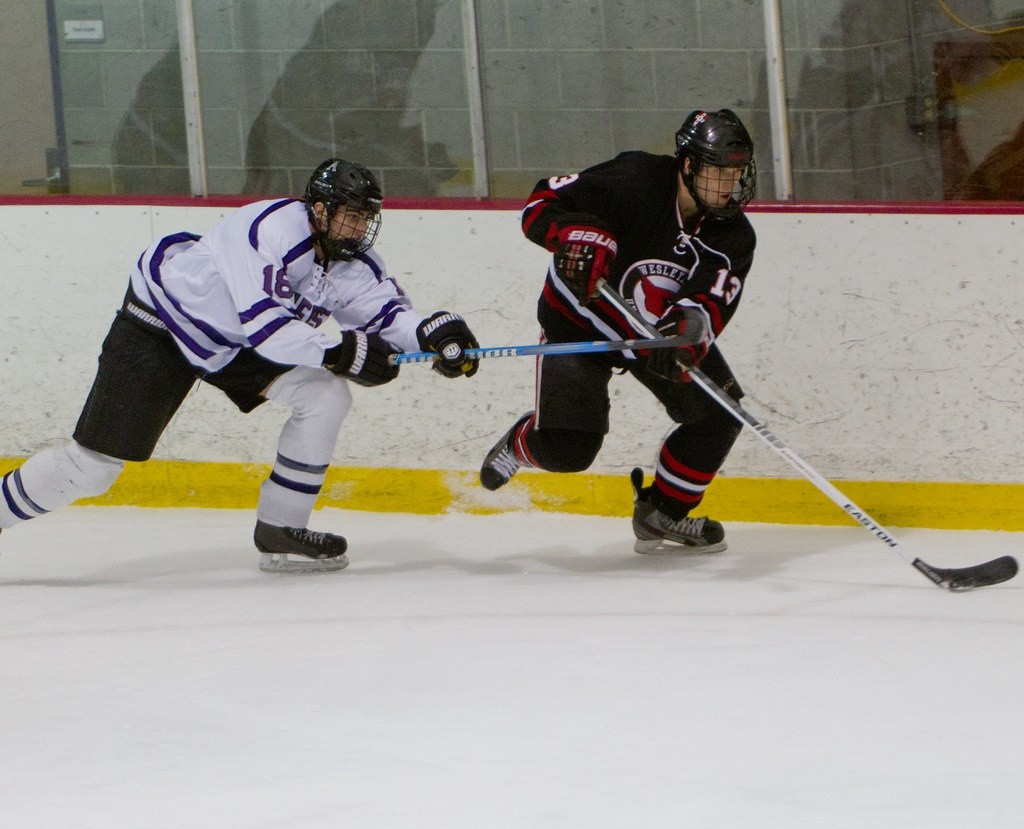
[554, 215, 619, 307]
[415, 311, 480, 378]
[644, 299, 715, 384]
[322, 327, 402, 386]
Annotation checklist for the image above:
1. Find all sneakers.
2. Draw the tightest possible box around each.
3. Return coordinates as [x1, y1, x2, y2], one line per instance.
[253, 520, 349, 573]
[632, 467, 728, 555]
[480, 411, 535, 491]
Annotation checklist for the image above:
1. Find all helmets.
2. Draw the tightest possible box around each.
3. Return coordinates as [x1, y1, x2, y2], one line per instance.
[304, 158, 385, 262]
[675, 108, 758, 222]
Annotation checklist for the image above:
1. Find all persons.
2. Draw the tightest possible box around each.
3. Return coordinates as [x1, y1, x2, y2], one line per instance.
[791, 1, 946, 204]
[477, 104, 759, 549]
[109, 1, 469, 202]
[0, 158, 483, 563]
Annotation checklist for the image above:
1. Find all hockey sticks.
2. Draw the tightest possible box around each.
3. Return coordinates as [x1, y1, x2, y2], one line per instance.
[596, 278, 1021, 593]
[388, 304, 711, 371]
